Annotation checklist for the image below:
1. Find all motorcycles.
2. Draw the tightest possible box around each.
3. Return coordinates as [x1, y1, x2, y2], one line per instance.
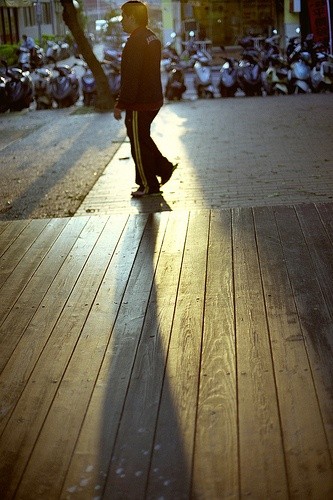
[1, 26, 333, 112]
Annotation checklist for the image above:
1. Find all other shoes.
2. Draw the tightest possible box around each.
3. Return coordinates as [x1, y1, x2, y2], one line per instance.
[161, 162, 178, 184]
[130, 180, 160, 195]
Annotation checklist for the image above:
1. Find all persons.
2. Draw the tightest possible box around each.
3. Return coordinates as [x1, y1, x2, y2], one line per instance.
[20, 34, 37, 70]
[113, 0, 179, 197]
